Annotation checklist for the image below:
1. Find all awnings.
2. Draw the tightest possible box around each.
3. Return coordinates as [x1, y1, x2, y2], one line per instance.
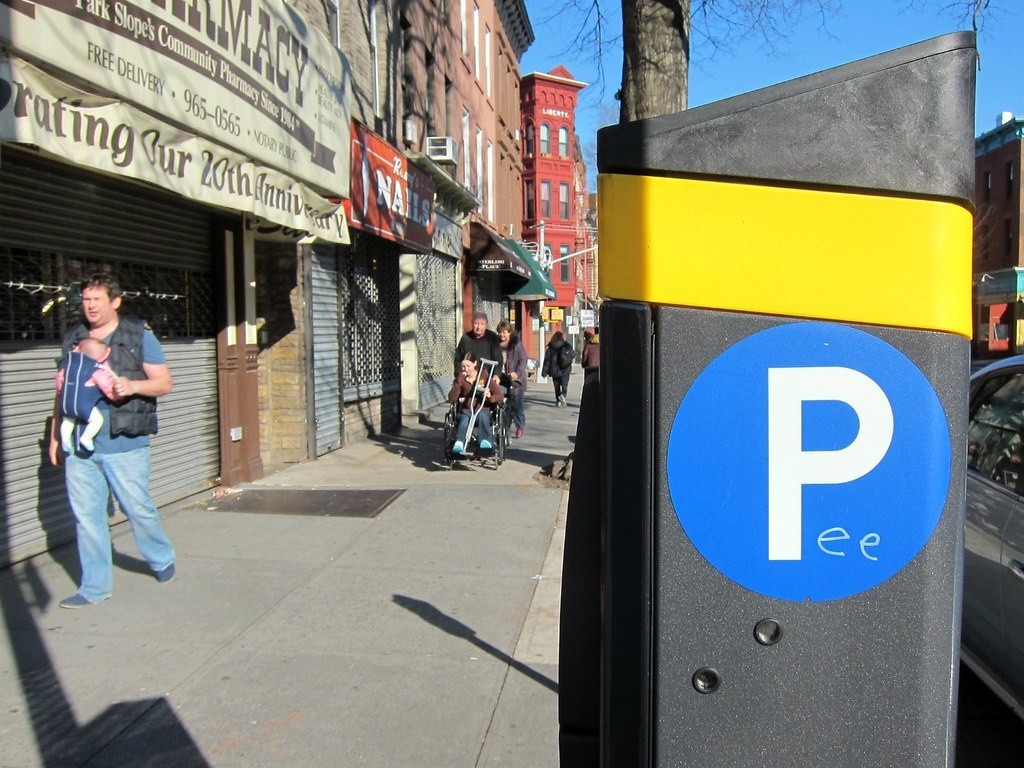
[503, 240, 558, 302]
[466, 222, 531, 280]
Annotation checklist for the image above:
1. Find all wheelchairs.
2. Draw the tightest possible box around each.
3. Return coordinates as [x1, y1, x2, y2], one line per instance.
[443, 373, 515, 471]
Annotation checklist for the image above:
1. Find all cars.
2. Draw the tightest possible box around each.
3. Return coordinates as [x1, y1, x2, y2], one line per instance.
[959, 354, 1024, 722]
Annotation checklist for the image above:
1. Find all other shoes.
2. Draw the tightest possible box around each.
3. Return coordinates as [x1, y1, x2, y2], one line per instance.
[516, 428, 523, 438]
[559, 395, 567, 407]
[480, 439, 492, 448]
[452, 441, 463, 453]
[556, 401, 561, 407]
[60, 594, 111, 609]
[157, 562, 175, 584]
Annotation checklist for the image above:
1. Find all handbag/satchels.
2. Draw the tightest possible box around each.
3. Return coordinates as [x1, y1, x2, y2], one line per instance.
[559, 343, 573, 368]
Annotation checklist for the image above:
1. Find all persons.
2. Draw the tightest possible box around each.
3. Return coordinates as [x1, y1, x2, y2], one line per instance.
[447, 353, 505, 453]
[580, 326, 600, 369]
[540, 332, 575, 409]
[54, 336, 122, 455]
[496, 318, 527, 438]
[453, 311, 512, 445]
[48, 273, 177, 609]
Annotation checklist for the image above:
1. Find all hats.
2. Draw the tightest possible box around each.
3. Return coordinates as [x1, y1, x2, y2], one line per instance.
[584, 328, 595, 336]
